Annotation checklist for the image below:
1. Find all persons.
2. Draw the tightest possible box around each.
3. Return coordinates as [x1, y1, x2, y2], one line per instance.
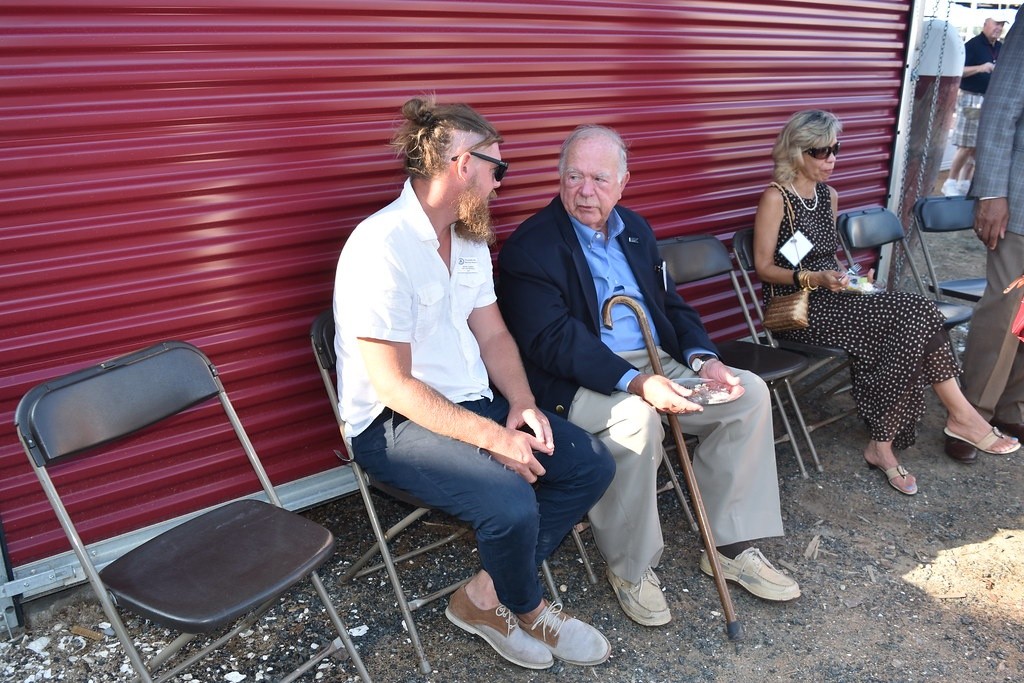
[494, 122, 800, 628]
[945, 2, 1024, 461]
[332, 98, 617, 671]
[940, 10, 1009, 196]
[754, 109, 1021, 494]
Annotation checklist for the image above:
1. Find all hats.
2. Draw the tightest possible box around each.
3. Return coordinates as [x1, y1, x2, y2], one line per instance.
[983, 11, 1009, 24]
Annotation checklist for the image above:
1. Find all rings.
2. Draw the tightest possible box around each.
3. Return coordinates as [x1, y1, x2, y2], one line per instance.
[977, 227, 983, 231]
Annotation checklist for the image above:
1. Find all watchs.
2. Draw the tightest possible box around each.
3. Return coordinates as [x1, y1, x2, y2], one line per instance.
[692, 354, 717, 375]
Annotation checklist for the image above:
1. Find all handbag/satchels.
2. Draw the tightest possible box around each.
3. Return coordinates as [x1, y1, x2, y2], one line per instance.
[761, 287, 809, 332]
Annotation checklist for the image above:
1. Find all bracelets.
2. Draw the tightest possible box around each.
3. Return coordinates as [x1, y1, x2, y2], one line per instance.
[793, 267, 818, 290]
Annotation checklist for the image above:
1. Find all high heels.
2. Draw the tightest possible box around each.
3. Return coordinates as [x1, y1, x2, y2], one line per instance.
[943, 418, 1021, 453]
[863, 449, 918, 495]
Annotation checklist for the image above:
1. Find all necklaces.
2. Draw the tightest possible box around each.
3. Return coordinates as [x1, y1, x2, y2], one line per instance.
[790, 182, 818, 210]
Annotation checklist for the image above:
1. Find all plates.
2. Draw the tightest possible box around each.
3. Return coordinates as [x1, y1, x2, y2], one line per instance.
[840, 277, 888, 295]
[664, 378, 746, 405]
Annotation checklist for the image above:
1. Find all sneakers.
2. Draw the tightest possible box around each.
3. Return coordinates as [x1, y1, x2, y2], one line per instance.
[699, 546, 801, 601]
[941, 181, 962, 197]
[445, 585, 554, 669]
[958, 180, 971, 196]
[605, 564, 671, 626]
[516, 598, 611, 666]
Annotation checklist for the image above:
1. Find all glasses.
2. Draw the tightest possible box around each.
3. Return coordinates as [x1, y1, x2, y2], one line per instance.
[803, 141, 840, 159]
[451, 152, 507, 181]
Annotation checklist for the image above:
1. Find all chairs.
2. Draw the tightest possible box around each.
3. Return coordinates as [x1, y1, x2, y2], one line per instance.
[15, 339, 375, 683]
[913, 195, 986, 364]
[835, 204, 974, 389]
[656, 233, 811, 482]
[576, 446, 699, 586]
[312, 307, 563, 675]
[732, 227, 859, 472]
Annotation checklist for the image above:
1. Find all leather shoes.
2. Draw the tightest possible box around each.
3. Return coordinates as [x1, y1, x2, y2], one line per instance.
[945, 436, 978, 464]
[989, 417, 1024, 446]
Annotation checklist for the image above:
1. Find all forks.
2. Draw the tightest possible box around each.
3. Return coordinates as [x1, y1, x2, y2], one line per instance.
[837, 263, 863, 282]
